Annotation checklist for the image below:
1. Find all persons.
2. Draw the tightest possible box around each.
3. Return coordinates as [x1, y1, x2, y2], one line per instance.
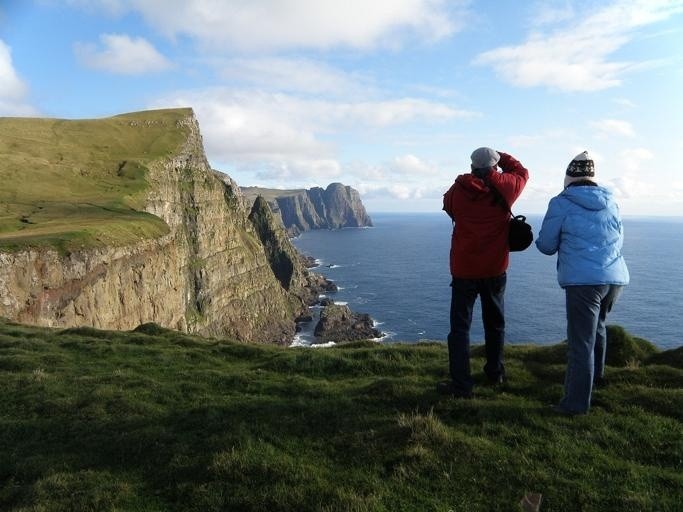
[535, 151, 630, 412]
[437, 147, 529, 394]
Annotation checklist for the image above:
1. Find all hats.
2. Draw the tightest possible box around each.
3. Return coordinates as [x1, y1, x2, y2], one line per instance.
[471, 146, 501, 169]
[563, 151, 595, 187]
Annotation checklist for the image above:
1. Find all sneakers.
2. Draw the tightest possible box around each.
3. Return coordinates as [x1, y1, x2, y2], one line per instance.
[486, 371, 505, 386]
[437, 379, 474, 397]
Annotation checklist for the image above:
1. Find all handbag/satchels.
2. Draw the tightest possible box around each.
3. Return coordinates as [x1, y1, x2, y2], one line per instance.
[509, 215, 533, 252]
